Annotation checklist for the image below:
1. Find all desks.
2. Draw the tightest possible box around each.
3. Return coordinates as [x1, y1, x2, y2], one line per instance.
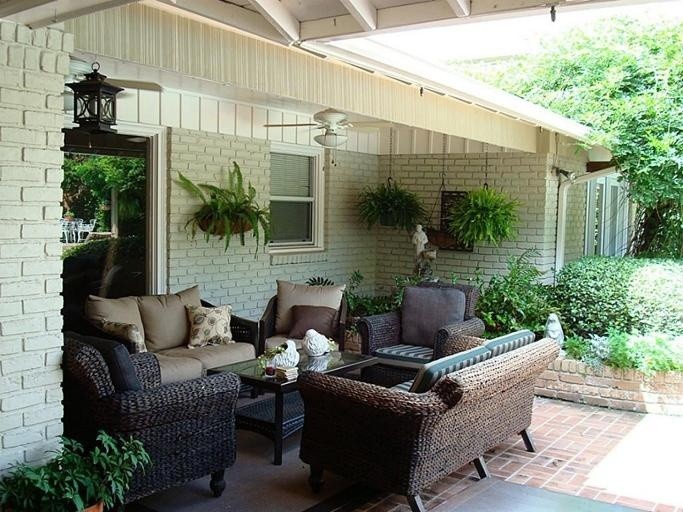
[208, 347, 380, 465]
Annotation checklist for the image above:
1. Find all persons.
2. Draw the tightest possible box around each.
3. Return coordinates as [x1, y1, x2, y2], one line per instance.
[411, 224, 428, 259]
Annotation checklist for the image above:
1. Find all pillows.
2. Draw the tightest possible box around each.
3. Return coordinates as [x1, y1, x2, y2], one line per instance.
[89, 317, 146, 354]
[290, 304, 336, 339]
[184, 305, 233, 349]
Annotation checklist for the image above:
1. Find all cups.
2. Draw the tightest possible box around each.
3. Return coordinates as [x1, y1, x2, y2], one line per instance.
[265, 358, 276, 377]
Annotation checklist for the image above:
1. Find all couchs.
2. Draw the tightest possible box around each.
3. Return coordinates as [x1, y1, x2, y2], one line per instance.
[79, 285, 257, 386]
[299, 329, 561, 512]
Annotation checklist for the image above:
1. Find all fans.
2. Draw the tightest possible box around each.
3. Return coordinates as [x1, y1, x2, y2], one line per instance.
[263, 106, 394, 170]
[63, 55, 164, 111]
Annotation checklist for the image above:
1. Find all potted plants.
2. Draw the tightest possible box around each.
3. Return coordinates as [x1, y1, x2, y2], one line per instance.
[171, 162, 271, 259]
[355, 176, 431, 237]
[1, 429, 154, 512]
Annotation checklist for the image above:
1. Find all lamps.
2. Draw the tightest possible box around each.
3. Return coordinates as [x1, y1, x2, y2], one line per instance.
[67, 54, 124, 133]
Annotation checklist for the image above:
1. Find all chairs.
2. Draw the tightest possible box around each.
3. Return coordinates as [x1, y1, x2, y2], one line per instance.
[359, 281, 485, 362]
[260, 280, 348, 354]
[62, 327, 242, 499]
